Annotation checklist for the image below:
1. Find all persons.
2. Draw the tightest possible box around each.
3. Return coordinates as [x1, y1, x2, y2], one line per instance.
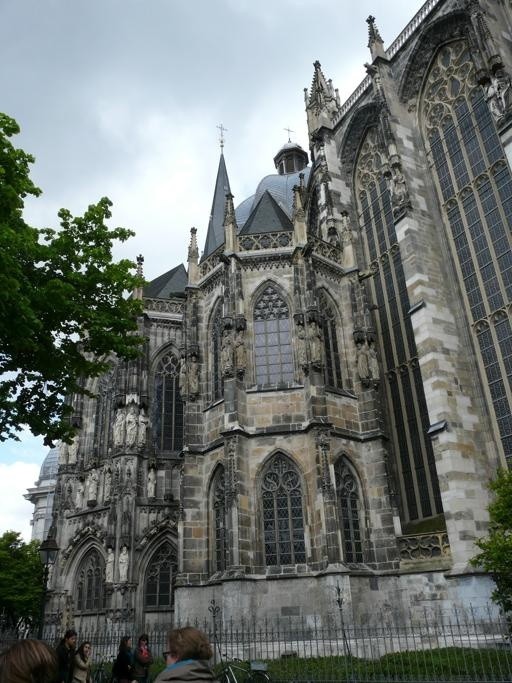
[133, 633, 153, 682]
[55, 628, 78, 682]
[296, 324, 307, 363]
[153, 626, 221, 682]
[369, 342, 380, 378]
[339, 209, 349, 232]
[178, 358, 187, 397]
[483, 82, 504, 123]
[392, 169, 406, 201]
[308, 321, 322, 361]
[72, 642, 94, 682]
[356, 342, 369, 380]
[220, 329, 234, 374]
[187, 356, 199, 393]
[115, 635, 137, 682]
[494, 69, 511, 109]
[233, 331, 248, 369]
[0, 638, 60, 682]
[48, 406, 158, 587]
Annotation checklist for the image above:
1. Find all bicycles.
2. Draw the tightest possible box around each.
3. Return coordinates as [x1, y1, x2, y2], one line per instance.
[216, 650, 273, 683]
[91, 651, 115, 683]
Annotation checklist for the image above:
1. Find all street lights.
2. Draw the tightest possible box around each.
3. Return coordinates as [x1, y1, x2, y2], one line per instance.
[36, 533, 59, 639]
